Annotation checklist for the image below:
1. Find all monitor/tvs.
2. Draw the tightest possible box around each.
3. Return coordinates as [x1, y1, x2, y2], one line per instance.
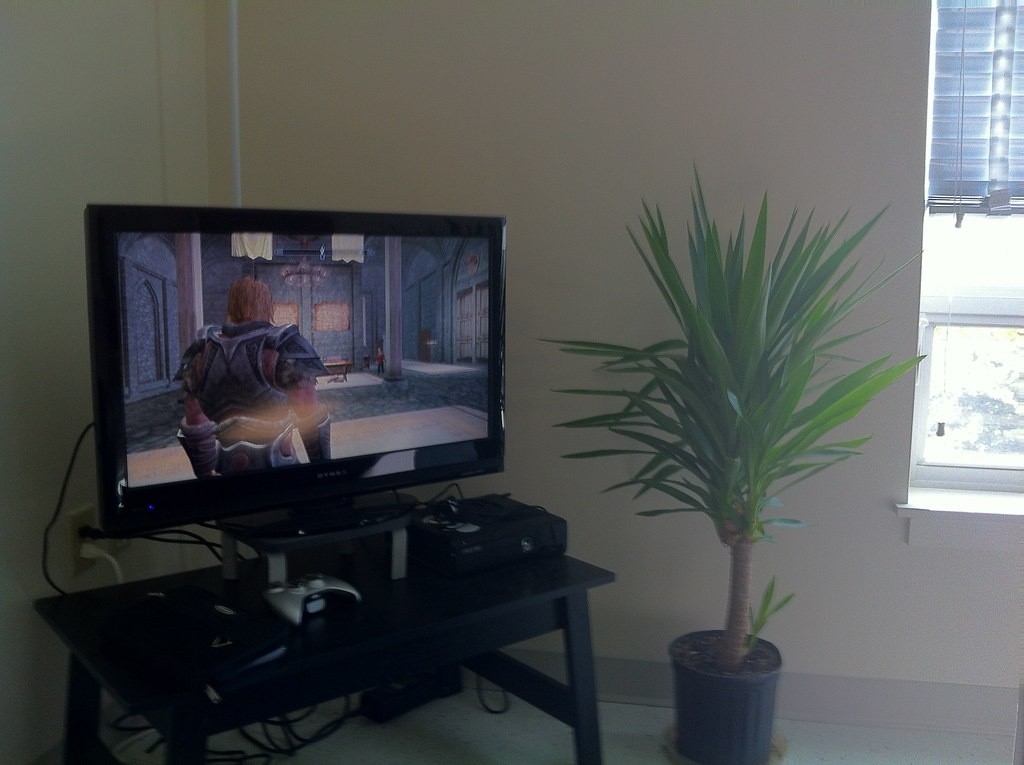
[85, 204, 508, 539]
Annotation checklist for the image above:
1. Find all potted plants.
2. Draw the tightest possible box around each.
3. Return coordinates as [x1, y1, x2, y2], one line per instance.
[536, 162, 926, 765]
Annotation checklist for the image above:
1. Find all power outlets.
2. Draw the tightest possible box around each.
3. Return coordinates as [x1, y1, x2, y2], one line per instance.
[68, 504, 103, 576]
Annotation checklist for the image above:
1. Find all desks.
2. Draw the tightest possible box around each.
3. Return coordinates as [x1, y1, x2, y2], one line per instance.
[34, 554, 615, 765]
[323, 361, 352, 382]
